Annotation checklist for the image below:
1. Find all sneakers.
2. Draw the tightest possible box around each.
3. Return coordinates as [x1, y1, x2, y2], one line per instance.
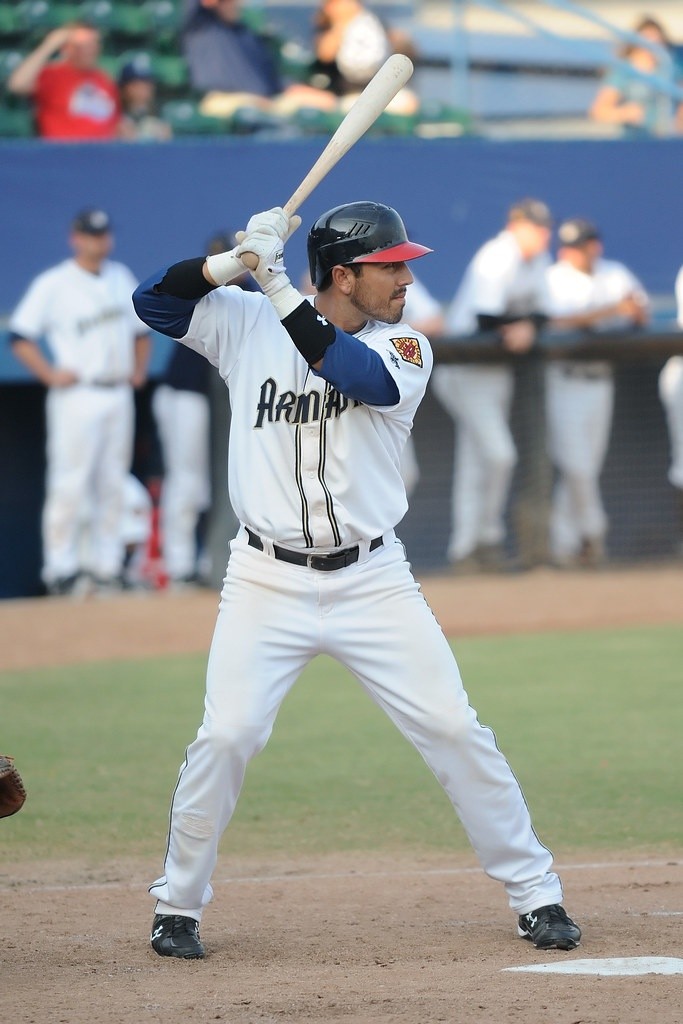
[517, 904, 581, 949]
[150, 914, 204, 959]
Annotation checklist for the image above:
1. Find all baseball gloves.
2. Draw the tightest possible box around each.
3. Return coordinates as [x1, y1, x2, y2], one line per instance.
[0, 754, 26, 819]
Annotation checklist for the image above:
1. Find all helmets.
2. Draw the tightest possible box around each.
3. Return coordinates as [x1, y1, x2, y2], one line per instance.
[307, 201, 434, 289]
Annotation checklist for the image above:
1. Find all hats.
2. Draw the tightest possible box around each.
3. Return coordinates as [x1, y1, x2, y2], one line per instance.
[75, 209, 110, 234]
[558, 218, 599, 245]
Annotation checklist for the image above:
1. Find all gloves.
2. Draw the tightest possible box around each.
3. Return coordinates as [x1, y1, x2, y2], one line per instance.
[206, 207, 301, 285]
[235, 226, 305, 320]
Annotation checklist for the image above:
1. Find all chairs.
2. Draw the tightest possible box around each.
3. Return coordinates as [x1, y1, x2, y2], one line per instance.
[0, 0, 474, 133]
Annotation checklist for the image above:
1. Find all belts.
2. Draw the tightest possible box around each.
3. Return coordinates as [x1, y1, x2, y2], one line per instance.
[244, 526, 383, 571]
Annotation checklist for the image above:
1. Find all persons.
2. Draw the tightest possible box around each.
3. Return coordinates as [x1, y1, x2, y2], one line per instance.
[430, 202, 553, 574]
[5, 207, 150, 596]
[591, 18, 683, 138]
[6, 0, 421, 142]
[657, 264, 683, 488]
[150, 232, 265, 588]
[540, 218, 654, 569]
[303, 268, 451, 503]
[132, 200, 581, 959]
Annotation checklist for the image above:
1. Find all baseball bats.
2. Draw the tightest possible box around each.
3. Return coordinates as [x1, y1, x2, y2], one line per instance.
[240, 52, 414, 271]
